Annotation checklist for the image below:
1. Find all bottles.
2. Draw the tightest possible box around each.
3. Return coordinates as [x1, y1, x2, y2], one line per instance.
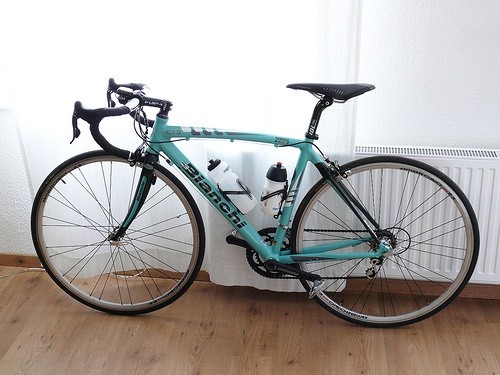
[208, 159, 258, 214]
[260, 162, 287, 215]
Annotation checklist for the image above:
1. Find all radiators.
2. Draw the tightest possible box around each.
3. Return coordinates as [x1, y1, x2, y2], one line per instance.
[347, 145, 500, 285]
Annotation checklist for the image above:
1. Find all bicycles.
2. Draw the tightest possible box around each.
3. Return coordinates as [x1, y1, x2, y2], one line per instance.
[31, 78, 480, 328]
